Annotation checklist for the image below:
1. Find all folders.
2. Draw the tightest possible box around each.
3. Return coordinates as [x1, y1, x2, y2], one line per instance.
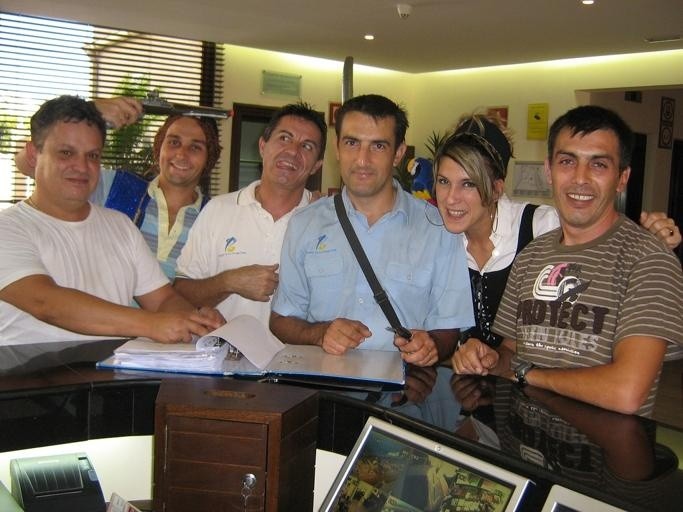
[94, 324, 406, 389]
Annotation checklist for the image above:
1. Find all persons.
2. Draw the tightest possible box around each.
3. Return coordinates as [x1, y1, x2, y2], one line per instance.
[175, 99, 327, 332]
[453, 104, 682, 415]
[270, 95, 475, 368]
[425, 108, 682, 382]
[0, 95, 226, 342]
[357, 371, 682, 508]
[16, 95, 224, 308]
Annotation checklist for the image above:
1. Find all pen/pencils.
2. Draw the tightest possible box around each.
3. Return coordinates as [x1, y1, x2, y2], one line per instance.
[395, 328, 413, 341]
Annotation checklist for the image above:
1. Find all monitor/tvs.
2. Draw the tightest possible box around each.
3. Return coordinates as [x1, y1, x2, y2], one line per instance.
[541, 484, 628, 512]
[319, 415, 536, 512]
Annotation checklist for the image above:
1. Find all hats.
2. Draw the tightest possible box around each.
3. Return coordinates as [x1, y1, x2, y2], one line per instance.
[446, 116, 512, 176]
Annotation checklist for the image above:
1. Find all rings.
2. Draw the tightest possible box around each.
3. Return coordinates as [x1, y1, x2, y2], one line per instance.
[667, 226, 675, 236]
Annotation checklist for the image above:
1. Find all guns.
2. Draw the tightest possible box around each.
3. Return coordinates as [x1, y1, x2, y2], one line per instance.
[104, 89, 234, 129]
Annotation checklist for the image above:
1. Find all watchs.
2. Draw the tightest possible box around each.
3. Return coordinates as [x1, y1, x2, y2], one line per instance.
[511, 361, 535, 383]
